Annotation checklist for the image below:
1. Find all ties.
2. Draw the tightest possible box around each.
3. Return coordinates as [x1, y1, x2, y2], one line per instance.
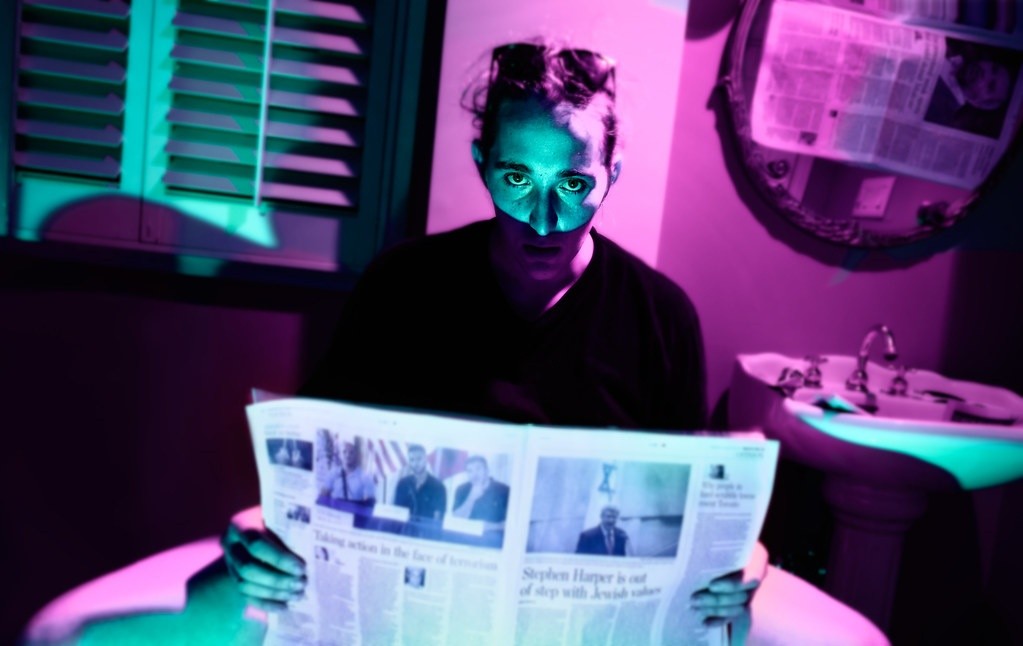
[607, 530, 613, 548]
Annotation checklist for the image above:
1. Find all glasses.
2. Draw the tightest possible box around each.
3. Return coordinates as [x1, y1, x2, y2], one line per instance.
[484, 42, 620, 99]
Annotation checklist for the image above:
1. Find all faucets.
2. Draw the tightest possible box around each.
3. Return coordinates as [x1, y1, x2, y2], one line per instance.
[845, 321, 899, 393]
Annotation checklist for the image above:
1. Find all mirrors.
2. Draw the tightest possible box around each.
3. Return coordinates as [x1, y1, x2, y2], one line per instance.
[718, 0, 1023, 267]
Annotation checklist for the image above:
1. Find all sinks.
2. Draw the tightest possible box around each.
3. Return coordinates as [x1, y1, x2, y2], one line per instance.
[735, 351, 1023, 489]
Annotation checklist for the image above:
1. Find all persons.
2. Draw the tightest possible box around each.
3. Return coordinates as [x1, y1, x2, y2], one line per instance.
[576, 506, 629, 556]
[452, 455, 510, 531]
[217, 40, 770, 646]
[320, 442, 376, 515]
[394, 444, 447, 524]
